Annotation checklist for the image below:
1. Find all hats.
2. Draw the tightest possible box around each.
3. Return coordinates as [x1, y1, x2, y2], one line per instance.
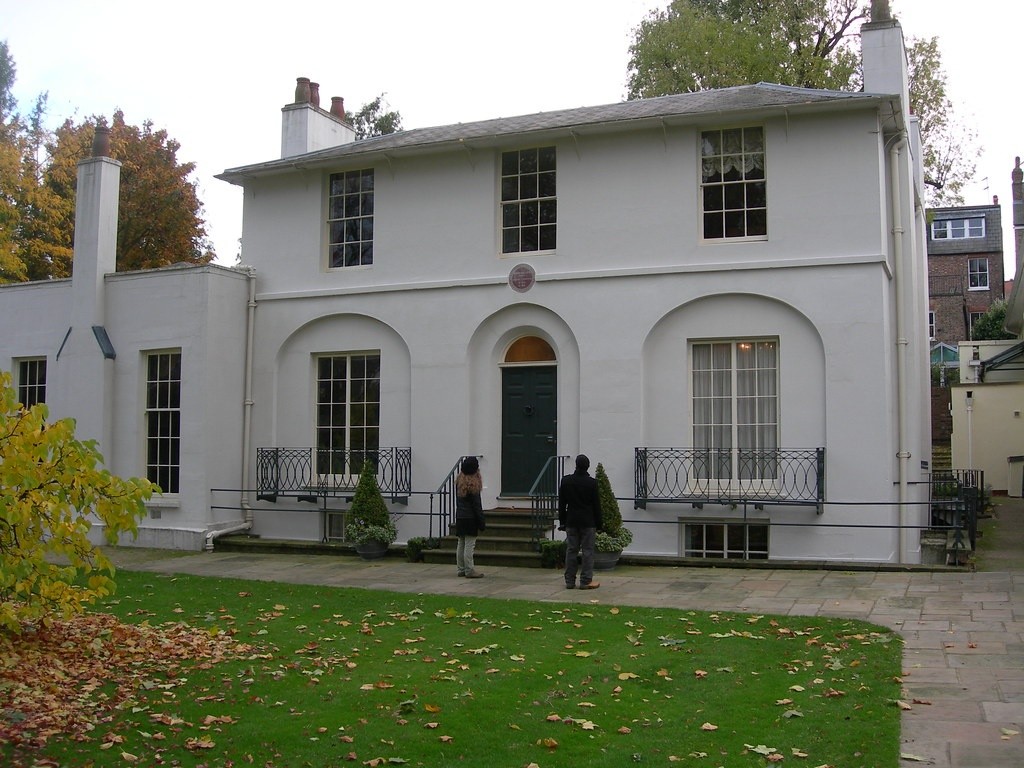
[461, 456, 479, 475]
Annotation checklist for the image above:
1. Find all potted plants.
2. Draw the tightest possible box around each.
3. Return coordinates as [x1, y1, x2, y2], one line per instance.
[343, 458, 399, 562]
[589, 463, 634, 570]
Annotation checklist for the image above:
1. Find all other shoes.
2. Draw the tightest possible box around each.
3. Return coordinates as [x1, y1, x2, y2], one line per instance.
[567, 582, 576, 589]
[580, 582, 600, 589]
[458, 570, 485, 578]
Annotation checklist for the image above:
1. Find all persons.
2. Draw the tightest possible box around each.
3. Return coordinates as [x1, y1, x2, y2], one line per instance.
[558, 454, 603, 589]
[454, 456, 486, 578]
